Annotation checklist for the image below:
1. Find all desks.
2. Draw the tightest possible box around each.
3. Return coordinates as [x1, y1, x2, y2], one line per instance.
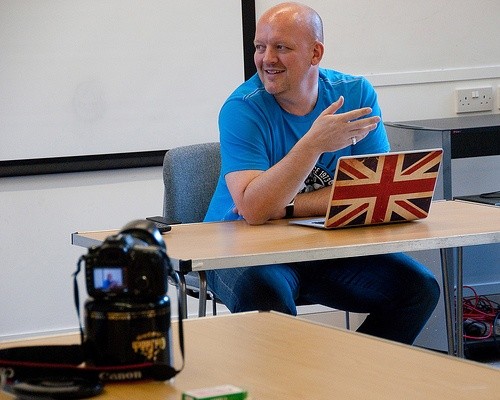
[0, 309, 500, 400]
[383, 113, 500, 356]
[72, 198, 500, 359]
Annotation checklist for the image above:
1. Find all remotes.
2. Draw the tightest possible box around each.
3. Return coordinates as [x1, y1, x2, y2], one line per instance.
[154, 222, 171, 232]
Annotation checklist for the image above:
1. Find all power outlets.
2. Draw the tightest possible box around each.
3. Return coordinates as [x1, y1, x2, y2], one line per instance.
[455, 87, 492, 114]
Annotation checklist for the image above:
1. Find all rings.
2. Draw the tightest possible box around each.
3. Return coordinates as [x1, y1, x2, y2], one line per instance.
[352, 137, 356, 146]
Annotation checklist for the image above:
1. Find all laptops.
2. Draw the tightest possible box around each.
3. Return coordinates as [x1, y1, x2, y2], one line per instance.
[288, 148, 443, 229]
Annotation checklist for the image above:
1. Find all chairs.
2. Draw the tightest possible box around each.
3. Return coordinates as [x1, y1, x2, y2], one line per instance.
[162, 141, 227, 316]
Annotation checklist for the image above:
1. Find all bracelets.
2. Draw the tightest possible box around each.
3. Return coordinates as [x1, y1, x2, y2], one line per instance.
[286, 198, 295, 218]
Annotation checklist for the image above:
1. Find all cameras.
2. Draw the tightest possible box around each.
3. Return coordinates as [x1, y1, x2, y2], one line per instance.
[85, 220, 173, 303]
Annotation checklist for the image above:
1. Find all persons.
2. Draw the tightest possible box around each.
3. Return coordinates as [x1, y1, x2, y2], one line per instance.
[203, 2, 440, 346]
[103, 274, 118, 289]
